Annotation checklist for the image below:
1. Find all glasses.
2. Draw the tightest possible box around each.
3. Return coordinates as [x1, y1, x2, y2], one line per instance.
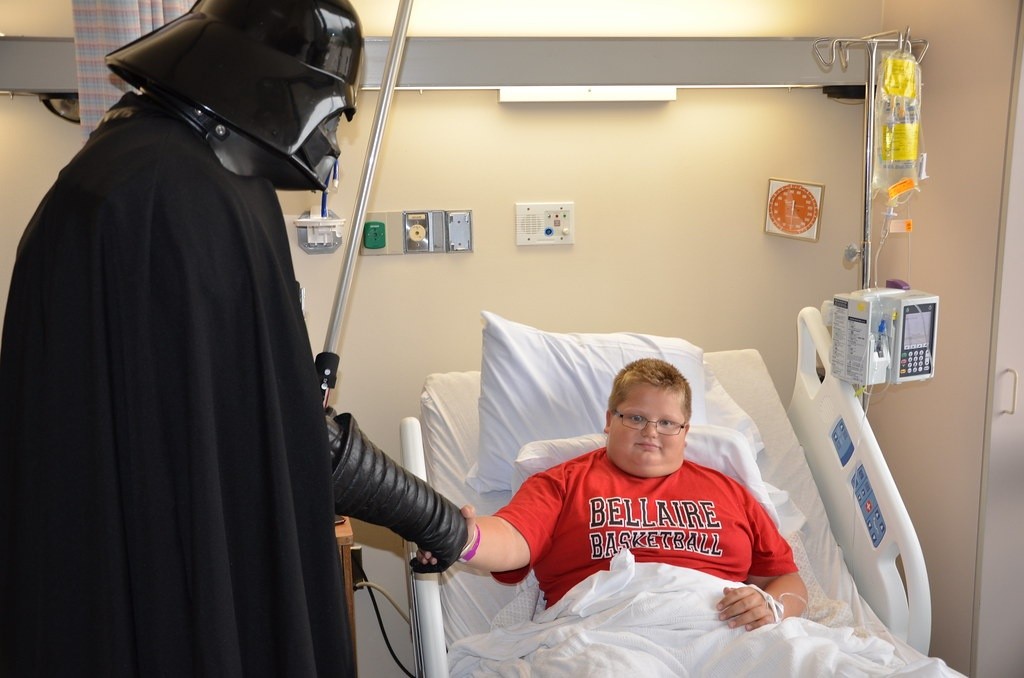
[613, 410, 687, 436]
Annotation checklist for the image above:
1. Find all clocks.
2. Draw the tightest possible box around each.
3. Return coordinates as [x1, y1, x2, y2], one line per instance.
[763, 176, 826, 243]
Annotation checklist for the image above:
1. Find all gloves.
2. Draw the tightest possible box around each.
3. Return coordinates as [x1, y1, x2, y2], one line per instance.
[337, 412, 468, 573]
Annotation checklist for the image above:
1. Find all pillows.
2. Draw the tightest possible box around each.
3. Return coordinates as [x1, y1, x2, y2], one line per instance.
[462, 310, 709, 496]
[511, 426, 781, 531]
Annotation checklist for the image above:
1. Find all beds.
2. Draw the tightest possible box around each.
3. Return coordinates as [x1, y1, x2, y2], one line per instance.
[399, 307, 976, 678]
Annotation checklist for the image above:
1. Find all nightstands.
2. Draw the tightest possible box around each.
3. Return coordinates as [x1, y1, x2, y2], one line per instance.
[334, 514, 358, 678]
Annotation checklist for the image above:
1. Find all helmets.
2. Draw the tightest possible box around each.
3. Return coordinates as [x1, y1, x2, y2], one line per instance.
[104, 1, 366, 192]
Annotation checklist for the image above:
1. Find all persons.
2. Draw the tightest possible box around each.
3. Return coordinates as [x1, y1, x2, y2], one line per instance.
[416, 358, 810, 632]
[1, 0, 467, 678]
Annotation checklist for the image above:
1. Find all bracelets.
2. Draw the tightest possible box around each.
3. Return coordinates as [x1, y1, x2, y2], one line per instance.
[457, 524, 481, 563]
